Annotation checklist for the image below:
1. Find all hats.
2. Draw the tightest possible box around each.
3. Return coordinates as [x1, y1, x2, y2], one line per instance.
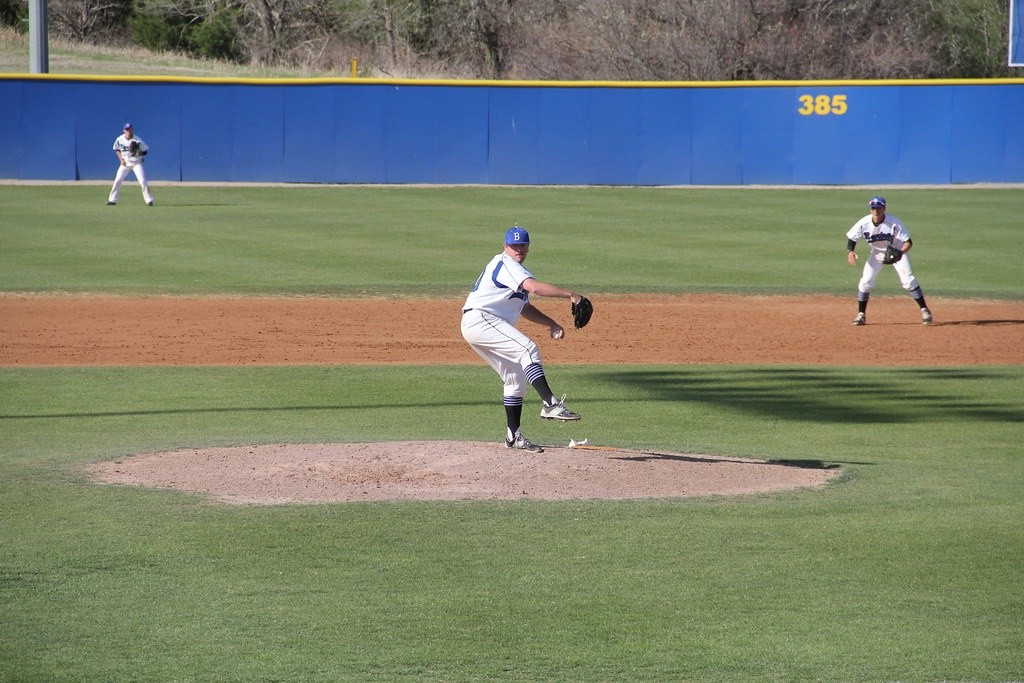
[123, 123, 133, 129]
[867, 196, 886, 208]
[505, 226, 531, 244]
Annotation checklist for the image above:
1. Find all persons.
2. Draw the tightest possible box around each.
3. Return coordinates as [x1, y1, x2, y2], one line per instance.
[460, 227, 595, 454]
[105, 123, 154, 208]
[843, 195, 934, 328]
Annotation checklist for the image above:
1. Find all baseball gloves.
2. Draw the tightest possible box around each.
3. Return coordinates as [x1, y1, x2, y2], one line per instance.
[572, 294, 593, 328]
[884, 246, 902, 264]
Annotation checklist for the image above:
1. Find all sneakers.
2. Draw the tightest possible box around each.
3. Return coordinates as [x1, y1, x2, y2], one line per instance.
[505, 433, 545, 453]
[921, 307, 932, 325]
[852, 312, 865, 326]
[147, 202, 153, 206]
[106, 201, 116, 205]
[540, 401, 582, 422]
